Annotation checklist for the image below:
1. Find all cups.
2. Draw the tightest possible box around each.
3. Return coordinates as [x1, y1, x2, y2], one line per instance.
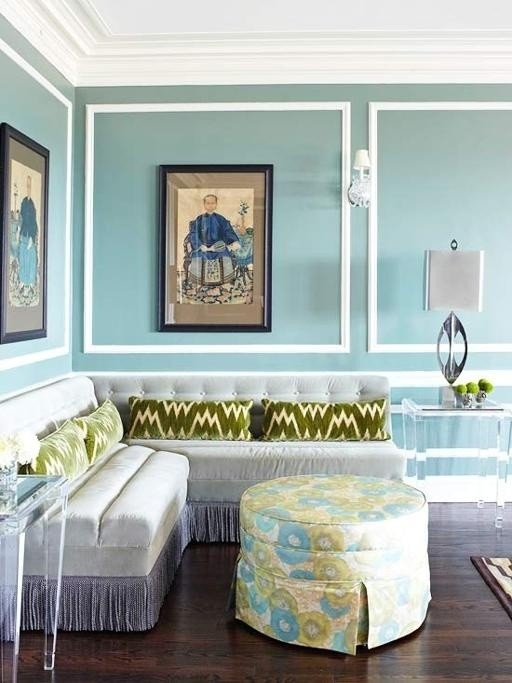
[457, 392, 474, 408]
[475, 390, 487, 404]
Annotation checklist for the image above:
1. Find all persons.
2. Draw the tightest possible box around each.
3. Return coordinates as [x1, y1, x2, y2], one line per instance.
[182, 193, 243, 295]
[13, 176, 39, 292]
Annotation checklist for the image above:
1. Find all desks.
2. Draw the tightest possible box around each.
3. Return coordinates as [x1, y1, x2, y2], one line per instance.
[225, 475, 431, 656]
[401, 398, 512, 528]
[0, 475, 71, 683]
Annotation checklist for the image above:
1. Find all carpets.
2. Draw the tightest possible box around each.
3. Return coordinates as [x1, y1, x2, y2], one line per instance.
[470, 555, 512, 620]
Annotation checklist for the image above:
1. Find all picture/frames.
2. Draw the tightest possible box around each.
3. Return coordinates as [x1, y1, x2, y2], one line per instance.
[1, 122, 49, 344]
[159, 165, 273, 332]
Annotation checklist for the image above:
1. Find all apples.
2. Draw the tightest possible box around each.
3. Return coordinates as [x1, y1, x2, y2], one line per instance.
[456, 378, 494, 394]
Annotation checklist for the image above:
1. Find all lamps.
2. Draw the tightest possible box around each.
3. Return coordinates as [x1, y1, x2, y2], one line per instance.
[423, 239, 485, 386]
[348, 149, 375, 208]
